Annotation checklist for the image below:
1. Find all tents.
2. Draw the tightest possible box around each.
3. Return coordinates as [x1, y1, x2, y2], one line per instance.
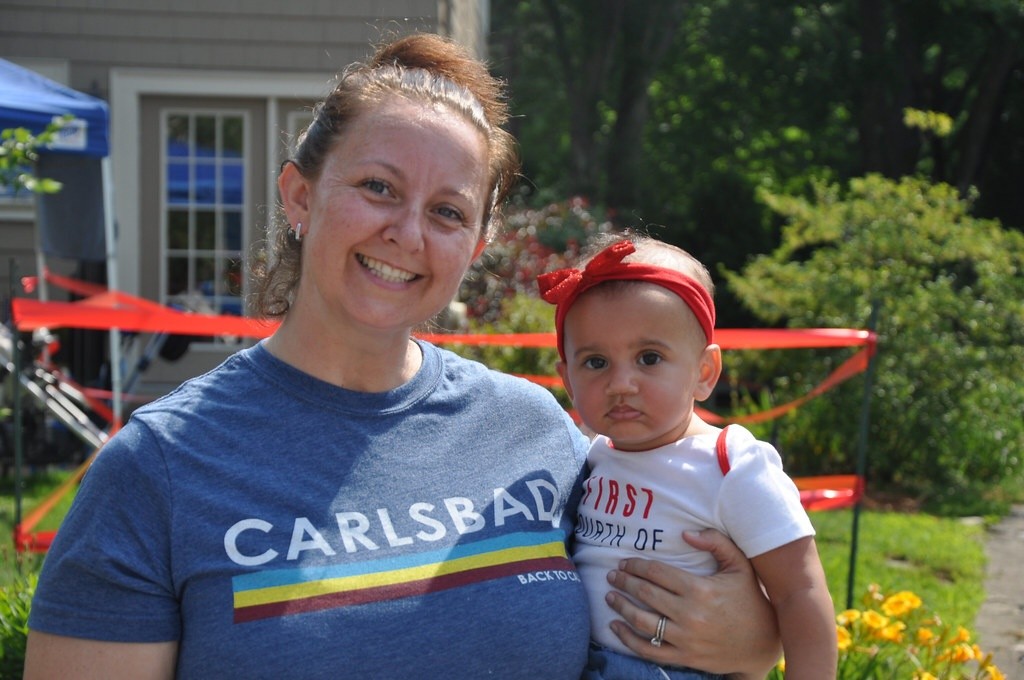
[169, 138, 242, 203]
[0, 57, 124, 522]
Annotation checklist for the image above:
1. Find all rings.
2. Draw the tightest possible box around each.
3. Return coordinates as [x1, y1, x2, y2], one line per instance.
[651, 616, 666, 647]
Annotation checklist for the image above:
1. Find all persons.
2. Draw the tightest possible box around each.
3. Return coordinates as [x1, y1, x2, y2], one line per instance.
[23, 33, 782, 680]
[537, 236, 836, 680]
